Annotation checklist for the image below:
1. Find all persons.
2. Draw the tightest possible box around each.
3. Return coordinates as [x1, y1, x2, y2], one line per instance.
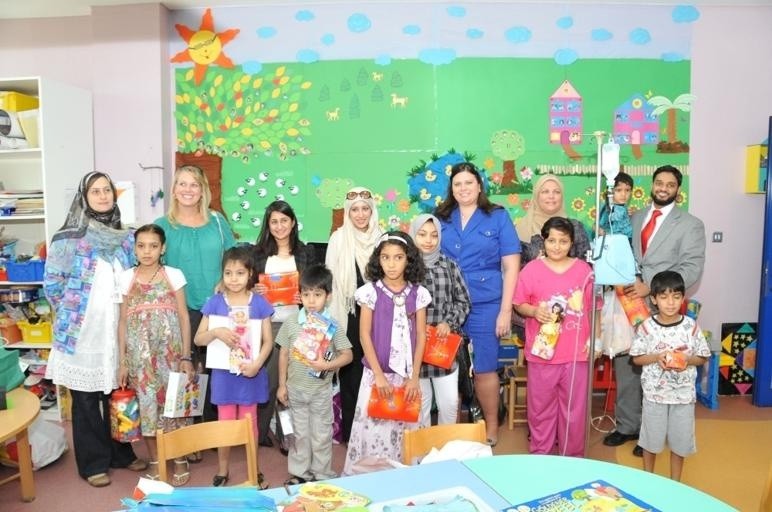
[434, 163, 523, 446]
[409, 213, 472, 428]
[324, 187, 387, 447]
[630, 270, 711, 483]
[602, 165, 706, 458]
[43, 171, 148, 487]
[342, 231, 432, 476]
[153, 165, 237, 373]
[246, 201, 317, 456]
[512, 216, 603, 459]
[592, 172, 633, 247]
[515, 174, 594, 272]
[117, 224, 192, 486]
[275, 266, 353, 485]
[194, 246, 273, 491]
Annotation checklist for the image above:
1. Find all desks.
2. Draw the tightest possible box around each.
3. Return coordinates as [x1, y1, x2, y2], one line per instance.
[0, 385, 40, 503]
[254, 453, 738, 512]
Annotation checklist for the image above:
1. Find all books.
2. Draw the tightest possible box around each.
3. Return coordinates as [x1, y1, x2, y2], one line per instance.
[366, 383, 422, 422]
[256, 272, 301, 307]
[421, 325, 463, 369]
[288, 311, 336, 369]
[1, 189, 43, 216]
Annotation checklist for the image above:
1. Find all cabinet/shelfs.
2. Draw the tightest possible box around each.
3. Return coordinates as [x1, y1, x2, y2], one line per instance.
[0, 75, 94, 423]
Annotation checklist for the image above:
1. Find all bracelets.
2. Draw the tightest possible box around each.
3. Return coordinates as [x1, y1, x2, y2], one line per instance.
[180, 354, 193, 363]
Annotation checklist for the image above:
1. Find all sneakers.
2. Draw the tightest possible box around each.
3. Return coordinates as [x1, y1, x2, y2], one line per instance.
[86, 471, 110, 487]
[125, 458, 149, 471]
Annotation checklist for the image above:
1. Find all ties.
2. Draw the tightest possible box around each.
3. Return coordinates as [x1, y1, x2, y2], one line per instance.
[640, 210, 662, 256]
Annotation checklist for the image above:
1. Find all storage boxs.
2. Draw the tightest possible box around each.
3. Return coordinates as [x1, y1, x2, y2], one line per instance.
[1, 320, 21, 346]
[15, 314, 51, 343]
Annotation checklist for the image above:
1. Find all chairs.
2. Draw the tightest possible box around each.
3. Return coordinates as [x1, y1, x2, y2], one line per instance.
[405, 421, 487, 466]
[155, 413, 258, 493]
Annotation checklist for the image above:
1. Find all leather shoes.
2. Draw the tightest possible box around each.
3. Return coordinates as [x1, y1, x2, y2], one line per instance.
[603, 431, 640, 446]
[633, 445, 643, 456]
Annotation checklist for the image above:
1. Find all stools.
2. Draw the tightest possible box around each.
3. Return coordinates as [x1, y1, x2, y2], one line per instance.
[503, 365, 532, 430]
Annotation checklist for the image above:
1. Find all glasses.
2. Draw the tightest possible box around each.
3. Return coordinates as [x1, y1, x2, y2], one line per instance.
[345, 190, 373, 200]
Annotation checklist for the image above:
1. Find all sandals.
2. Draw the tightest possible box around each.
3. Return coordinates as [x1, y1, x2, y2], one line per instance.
[146, 460, 161, 483]
[257, 472, 269, 491]
[170, 457, 191, 487]
[211, 469, 231, 489]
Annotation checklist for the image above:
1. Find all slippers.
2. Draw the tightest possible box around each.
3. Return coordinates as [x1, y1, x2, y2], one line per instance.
[187, 451, 203, 464]
